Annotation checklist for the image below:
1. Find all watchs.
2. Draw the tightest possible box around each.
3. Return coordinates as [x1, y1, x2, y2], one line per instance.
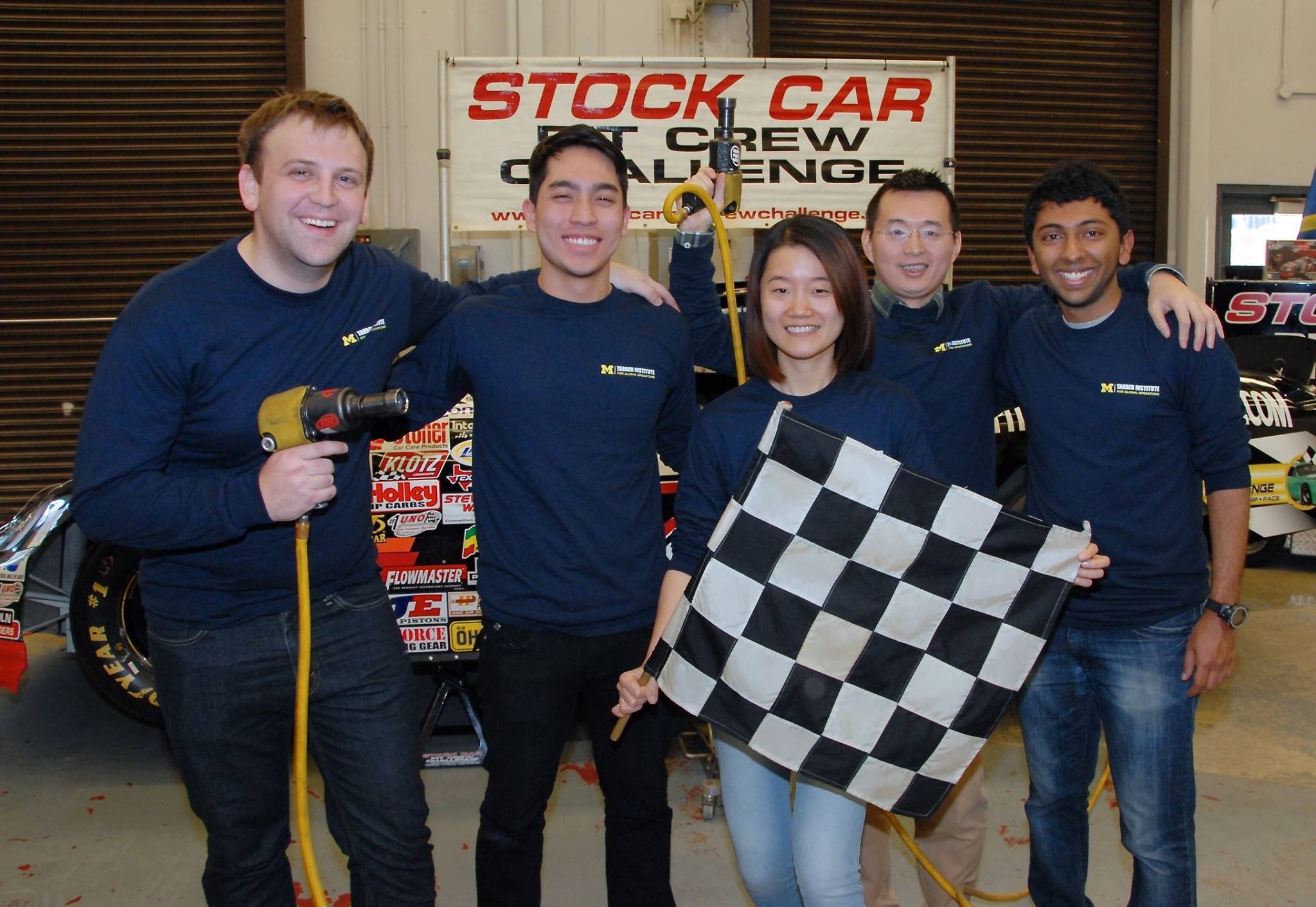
[1201, 596, 1249, 631]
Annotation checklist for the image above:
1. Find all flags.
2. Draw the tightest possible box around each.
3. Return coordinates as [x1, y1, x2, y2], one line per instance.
[642, 401, 1097, 825]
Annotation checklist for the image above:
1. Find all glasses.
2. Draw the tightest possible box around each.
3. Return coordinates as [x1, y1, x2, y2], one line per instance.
[870, 226, 954, 242]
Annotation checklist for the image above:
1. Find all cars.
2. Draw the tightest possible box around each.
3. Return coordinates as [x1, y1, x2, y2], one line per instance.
[0, 390, 683, 730]
[1201, 377, 1316, 566]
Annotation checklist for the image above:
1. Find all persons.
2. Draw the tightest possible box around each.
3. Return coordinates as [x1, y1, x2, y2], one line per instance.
[978, 164, 1251, 907]
[610, 212, 1111, 905]
[71, 86, 679, 906]
[667, 165, 1225, 906]
[395, 127, 698, 906]
